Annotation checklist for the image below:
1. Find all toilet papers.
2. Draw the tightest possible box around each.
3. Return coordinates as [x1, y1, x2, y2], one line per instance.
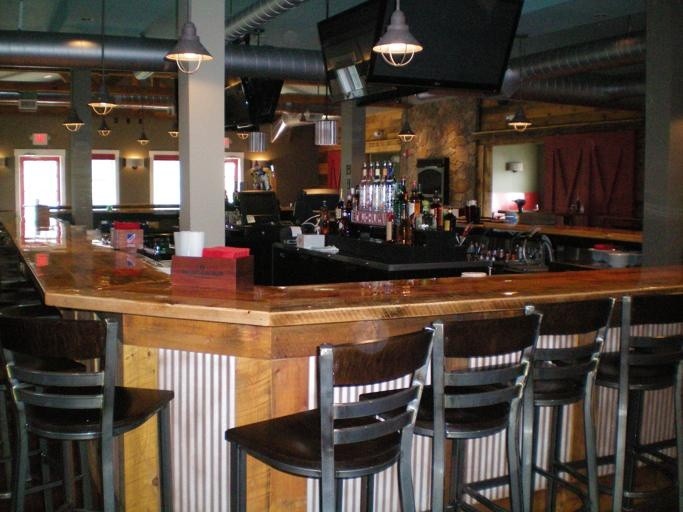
[172, 231, 205, 257]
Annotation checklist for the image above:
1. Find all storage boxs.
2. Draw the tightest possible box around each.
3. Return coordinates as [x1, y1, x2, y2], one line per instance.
[171, 254, 255, 291]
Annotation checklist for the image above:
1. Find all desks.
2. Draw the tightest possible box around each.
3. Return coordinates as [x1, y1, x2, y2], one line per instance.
[0, 207, 681, 511]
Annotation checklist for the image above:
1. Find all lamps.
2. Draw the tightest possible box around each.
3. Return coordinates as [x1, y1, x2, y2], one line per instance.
[167, 130, 180, 139]
[246, 130, 268, 155]
[94, 121, 112, 139]
[506, 34, 533, 134]
[236, 129, 248, 141]
[505, 161, 524, 172]
[372, 0, 424, 67]
[87, 1, 118, 118]
[163, 1, 215, 77]
[0, 156, 10, 167]
[61, 69, 86, 135]
[134, 80, 152, 147]
[398, 95, 418, 143]
[312, 116, 338, 148]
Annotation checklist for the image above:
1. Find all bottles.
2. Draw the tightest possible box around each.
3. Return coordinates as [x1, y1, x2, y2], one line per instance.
[318, 157, 457, 238]
[224, 190, 228, 204]
[462, 238, 522, 263]
[107, 205, 112, 212]
[464, 198, 479, 223]
[141, 237, 171, 255]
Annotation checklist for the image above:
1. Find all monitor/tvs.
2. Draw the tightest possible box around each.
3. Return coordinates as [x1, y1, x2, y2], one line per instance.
[224, 76, 259, 132]
[355, 86, 433, 107]
[317, 0, 407, 103]
[366, 0, 525, 96]
[250, 78, 284, 125]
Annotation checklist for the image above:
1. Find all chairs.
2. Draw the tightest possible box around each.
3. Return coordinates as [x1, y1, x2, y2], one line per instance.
[525, 296, 616, 511]
[224, 326, 436, 512]
[359, 310, 546, 512]
[2, 310, 174, 512]
[545, 293, 682, 512]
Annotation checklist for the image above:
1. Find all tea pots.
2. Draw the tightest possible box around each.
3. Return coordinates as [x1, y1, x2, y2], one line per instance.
[505, 228, 555, 273]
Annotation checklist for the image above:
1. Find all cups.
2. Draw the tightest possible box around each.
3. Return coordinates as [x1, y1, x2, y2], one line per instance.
[587, 245, 629, 269]
[260, 182, 264, 189]
